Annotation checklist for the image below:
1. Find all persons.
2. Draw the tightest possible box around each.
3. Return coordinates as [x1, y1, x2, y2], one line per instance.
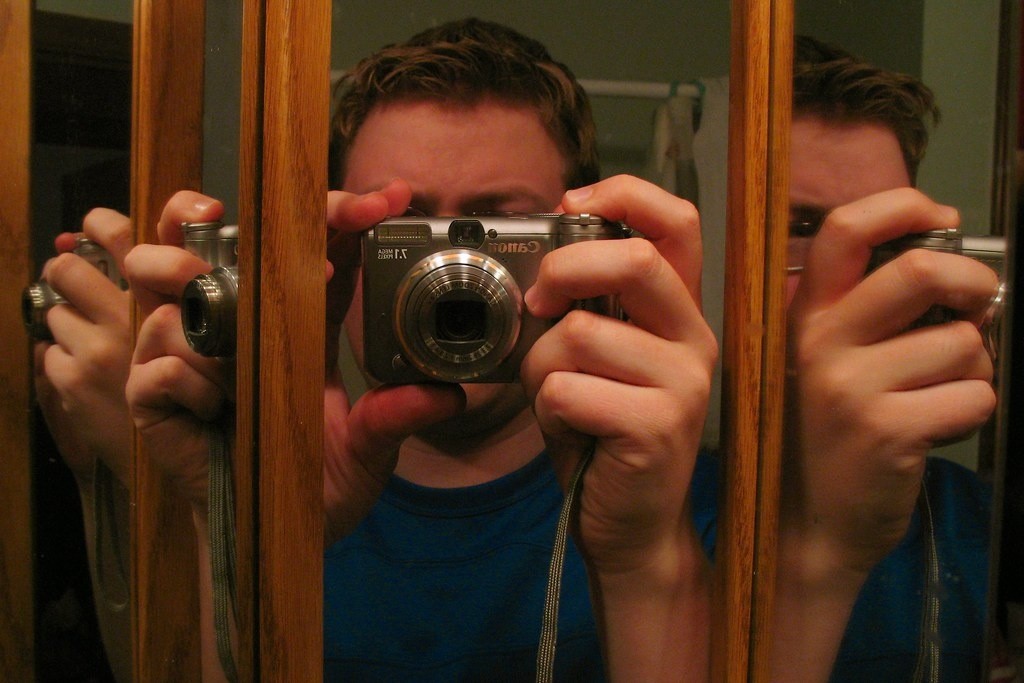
[778, 34, 1000, 682]
[22, 200, 133, 680]
[313, 18, 714, 681]
[123, 189, 241, 681]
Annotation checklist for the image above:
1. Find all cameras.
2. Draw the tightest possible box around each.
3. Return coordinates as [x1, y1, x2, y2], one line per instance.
[20, 238, 128, 345]
[863, 227, 1006, 385]
[180, 220, 242, 383]
[360, 211, 631, 385]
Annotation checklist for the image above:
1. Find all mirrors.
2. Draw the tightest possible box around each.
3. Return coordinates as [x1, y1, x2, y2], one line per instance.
[0, 0, 1024, 683]
